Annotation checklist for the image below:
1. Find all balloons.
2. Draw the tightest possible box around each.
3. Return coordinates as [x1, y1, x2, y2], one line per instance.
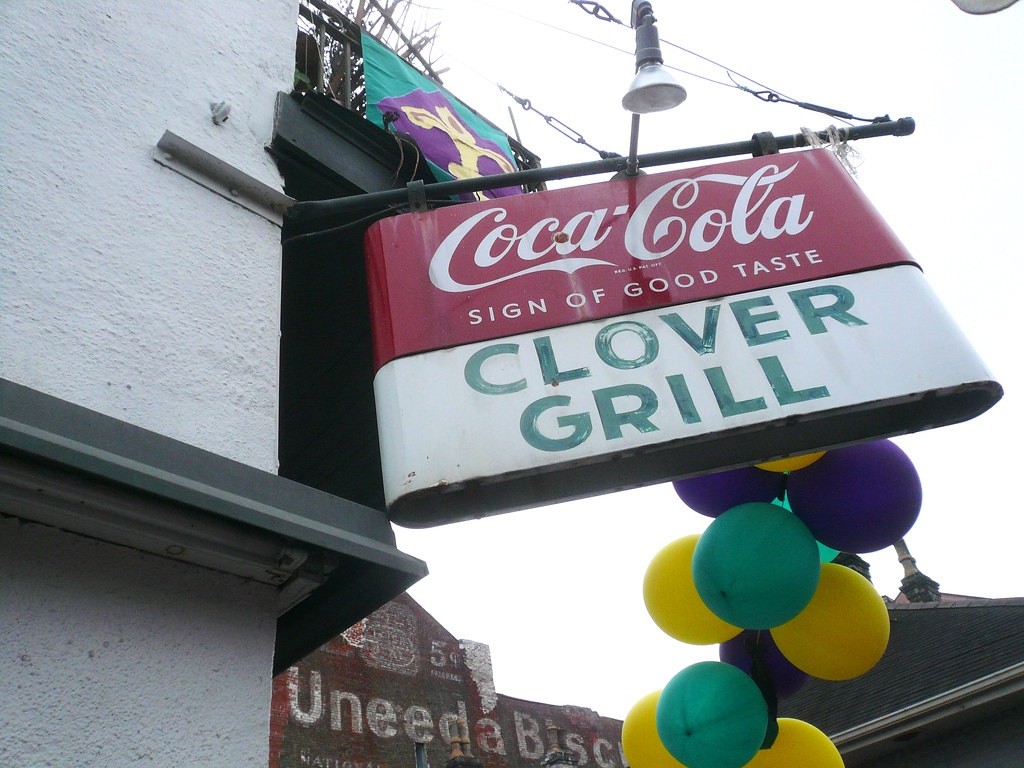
[643, 534, 746, 645]
[787, 440, 923, 554]
[743, 718, 845, 768]
[771, 490, 841, 563]
[754, 451, 826, 472]
[655, 661, 769, 768]
[692, 502, 820, 630]
[621, 690, 687, 768]
[770, 563, 890, 681]
[672, 466, 787, 518]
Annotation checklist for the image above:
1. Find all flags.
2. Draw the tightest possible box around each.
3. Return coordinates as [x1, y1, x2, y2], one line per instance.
[360, 28, 522, 201]
